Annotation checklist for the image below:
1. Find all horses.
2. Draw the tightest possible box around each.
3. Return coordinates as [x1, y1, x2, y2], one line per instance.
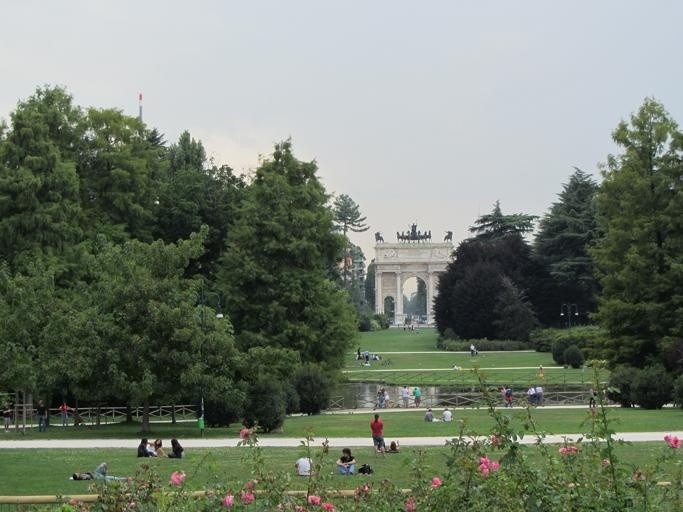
[375, 233, 384, 244]
[397, 230, 432, 244]
[444, 232, 452, 243]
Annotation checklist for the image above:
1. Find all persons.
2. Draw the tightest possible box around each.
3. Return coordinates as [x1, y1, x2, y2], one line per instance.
[137, 437, 157, 456]
[145, 438, 168, 458]
[293, 451, 317, 476]
[71, 461, 134, 481]
[589, 387, 598, 408]
[57, 401, 73, 427]
[35, 398, 48, 432]
[335, 344, 545, 476]
[166, 438, 184, 458]
[1, 401, 11, 432]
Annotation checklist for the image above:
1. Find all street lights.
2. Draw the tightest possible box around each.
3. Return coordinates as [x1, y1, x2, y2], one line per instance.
[559, 301, 578, 367]
[199, 284, 225, 437]
